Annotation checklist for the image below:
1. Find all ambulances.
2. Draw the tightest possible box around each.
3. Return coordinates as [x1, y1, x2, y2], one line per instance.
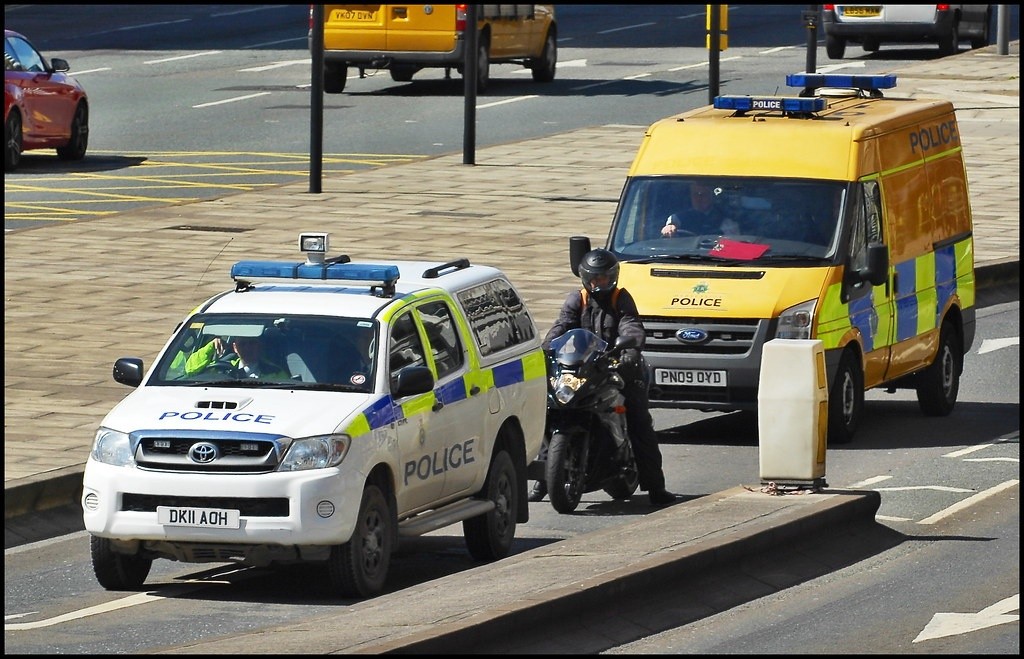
[569, 71, 977, 444]
[81, 227, 549, 600]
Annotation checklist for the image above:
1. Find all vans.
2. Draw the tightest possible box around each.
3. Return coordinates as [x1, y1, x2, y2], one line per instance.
[308, 3, 559, 95]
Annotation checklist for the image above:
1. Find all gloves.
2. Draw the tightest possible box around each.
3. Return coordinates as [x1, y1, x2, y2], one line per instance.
[620, 348, 639, 368]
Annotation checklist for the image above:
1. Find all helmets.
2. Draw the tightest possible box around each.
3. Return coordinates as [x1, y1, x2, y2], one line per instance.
[577, 250, 620, 297]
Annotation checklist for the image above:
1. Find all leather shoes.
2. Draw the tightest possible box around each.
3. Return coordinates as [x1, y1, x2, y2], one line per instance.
[528, 478, 549, 501]
[648, 488, 676, 503]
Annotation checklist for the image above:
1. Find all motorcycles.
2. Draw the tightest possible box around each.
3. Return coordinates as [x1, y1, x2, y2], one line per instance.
[526, 327, 643, 512]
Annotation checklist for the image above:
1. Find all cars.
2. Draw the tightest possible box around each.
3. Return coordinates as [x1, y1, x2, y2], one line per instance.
[3, 29, 89, 171]
[818, 2, 994, 62]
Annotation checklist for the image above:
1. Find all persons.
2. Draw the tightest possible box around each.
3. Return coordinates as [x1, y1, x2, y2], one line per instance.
[528, 248, 677, 506]
[183, 330, 377, 387]
[661, 184, 738, 240]
